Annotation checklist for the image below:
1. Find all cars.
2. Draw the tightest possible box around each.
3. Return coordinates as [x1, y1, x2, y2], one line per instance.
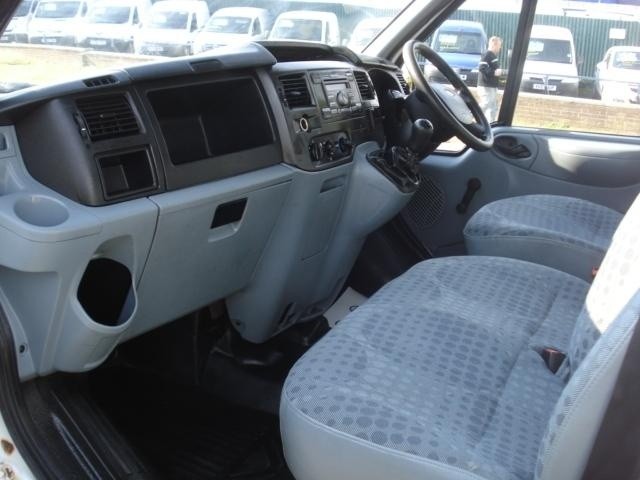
[346, 15, 394, 59]
[594, 45, 640, 106]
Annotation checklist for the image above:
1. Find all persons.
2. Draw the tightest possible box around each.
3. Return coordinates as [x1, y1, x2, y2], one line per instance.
[476, 36, 503, 123]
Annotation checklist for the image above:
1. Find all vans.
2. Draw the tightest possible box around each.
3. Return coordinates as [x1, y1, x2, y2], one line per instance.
[424, 20, 499, 87]
[506, 24, 582, 99]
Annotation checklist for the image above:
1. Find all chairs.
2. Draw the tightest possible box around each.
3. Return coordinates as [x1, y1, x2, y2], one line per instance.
[279, 191, 640, 480]
[463, 194, 627, 282]
